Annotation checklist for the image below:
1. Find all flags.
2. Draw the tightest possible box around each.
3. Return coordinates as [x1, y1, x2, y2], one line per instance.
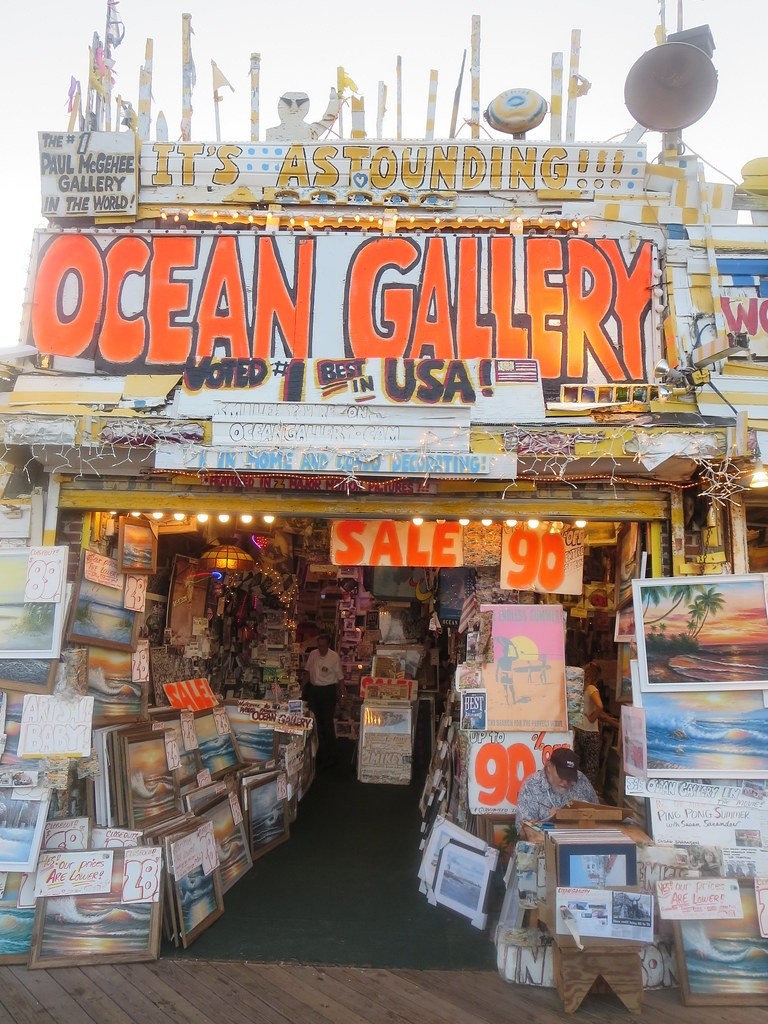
[212, 64, 234, 92]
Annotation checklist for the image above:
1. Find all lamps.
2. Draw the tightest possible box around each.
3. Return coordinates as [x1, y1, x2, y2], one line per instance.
[652, 358, 671, 379]
[749, 459, 768, 488]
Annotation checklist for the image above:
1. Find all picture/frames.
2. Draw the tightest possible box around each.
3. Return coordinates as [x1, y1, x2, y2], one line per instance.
[2, 493, 321, 970]
[629, 658, 767, 780]
[631, 572, 768, 693]
[327, 564, 445, 784]
[419, 568, 660, 945]
[665, 876, 767, 1008]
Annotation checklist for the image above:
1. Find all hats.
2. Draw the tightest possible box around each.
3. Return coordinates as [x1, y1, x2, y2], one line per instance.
[550, 747, 580, 781]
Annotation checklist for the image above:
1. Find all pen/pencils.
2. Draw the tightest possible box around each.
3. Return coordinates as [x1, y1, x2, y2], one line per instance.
[538, 802, 552, 809]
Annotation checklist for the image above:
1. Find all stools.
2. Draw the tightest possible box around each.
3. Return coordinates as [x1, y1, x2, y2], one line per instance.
[551, 930, 644, 1017]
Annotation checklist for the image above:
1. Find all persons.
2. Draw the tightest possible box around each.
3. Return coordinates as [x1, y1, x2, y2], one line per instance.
[440, 649, 454, 675]
[571, 662, 603, 785]
[515, 748, 599, 836]
[304, 635, 346, 768]
